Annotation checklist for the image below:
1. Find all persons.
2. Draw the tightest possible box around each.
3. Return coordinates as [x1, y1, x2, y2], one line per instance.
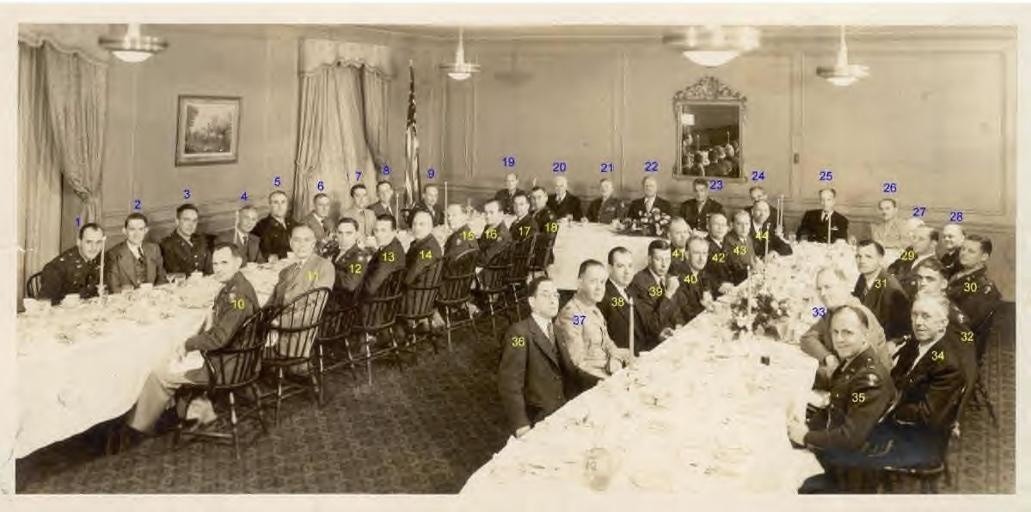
[682, 133, 739, 176]
[184, 106, 232, 153]
[38, 155, 1003, 494]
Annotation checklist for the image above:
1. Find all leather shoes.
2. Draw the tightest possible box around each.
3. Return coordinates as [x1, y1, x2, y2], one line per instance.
[179, 416, 219, 435]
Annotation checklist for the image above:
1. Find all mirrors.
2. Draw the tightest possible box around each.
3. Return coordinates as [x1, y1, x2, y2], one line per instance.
[670, 72, 748, 185]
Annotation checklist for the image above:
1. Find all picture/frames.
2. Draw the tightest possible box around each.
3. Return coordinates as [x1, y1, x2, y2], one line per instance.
[174, 94, 240, 167]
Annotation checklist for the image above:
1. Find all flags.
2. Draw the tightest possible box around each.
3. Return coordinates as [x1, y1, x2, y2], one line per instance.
[403, 63, 419, 224]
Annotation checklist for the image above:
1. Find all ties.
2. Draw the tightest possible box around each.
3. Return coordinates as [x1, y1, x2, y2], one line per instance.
[243, 236, 249, 255]
[557, 197, 562, 206]
[137, 247, 146, 280]
[823, 214, 828, 223]
[507, 193, 512, 203]
[385, 205, 392, 215]
[645, 200, 652, 213]
[547, 323, 556, 344]
[659, 278, 668, 296]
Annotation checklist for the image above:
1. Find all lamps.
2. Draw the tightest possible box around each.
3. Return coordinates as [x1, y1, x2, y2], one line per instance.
[96, 19, 171, 65]
[438, 24, 483, 82]
[654, 24, 763, 70]
[814, 23, 874, 87]
